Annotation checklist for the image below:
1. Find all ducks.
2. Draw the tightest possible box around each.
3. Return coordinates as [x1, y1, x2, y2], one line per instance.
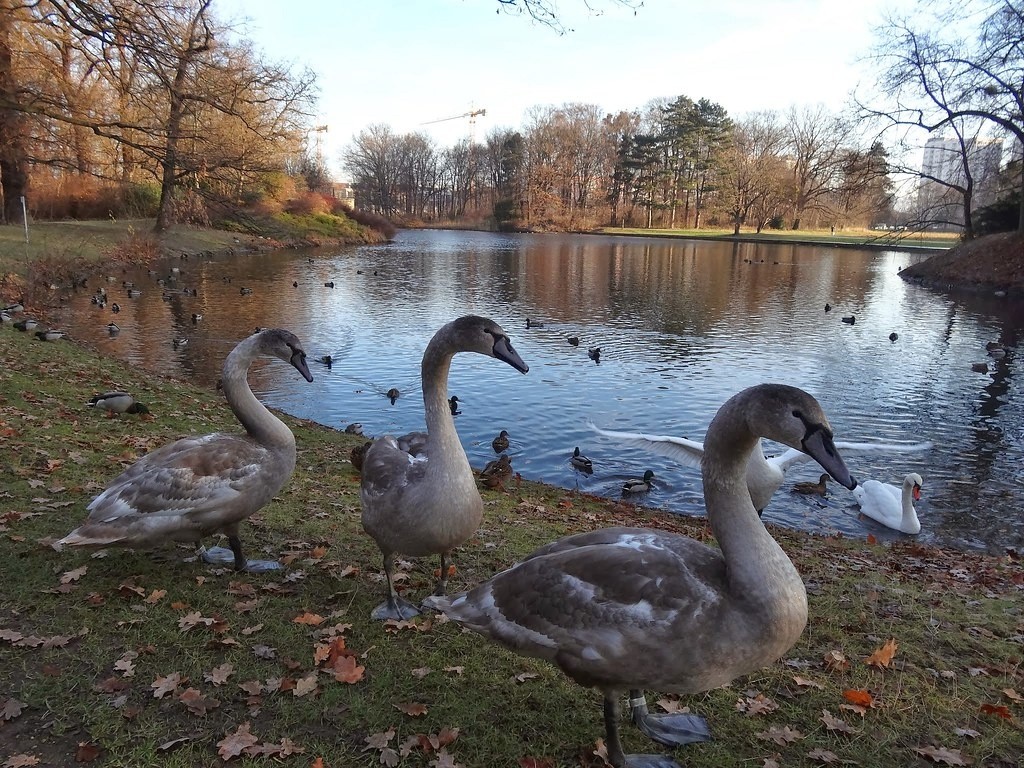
[86, 391, 155, 420]
[525, 317, 603, 361]
[386, 388, 400, 407]
[824, 303, 898, 343]
[321, 355, 336, 369]
[0, 267, 203, 348]
[478, 430, 515, 488]
[570, 445, 658, 494]
[223, 258, 383, 296]
[791, 473, 834, 497]
[446, 396, 462, 416]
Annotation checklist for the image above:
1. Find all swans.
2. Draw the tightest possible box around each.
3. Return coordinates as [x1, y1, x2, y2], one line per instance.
[421, 382, 857, 766]
[586, 420, 935, 536]
[57, 328, 315, 576]
[349, 314, 530, 621]
